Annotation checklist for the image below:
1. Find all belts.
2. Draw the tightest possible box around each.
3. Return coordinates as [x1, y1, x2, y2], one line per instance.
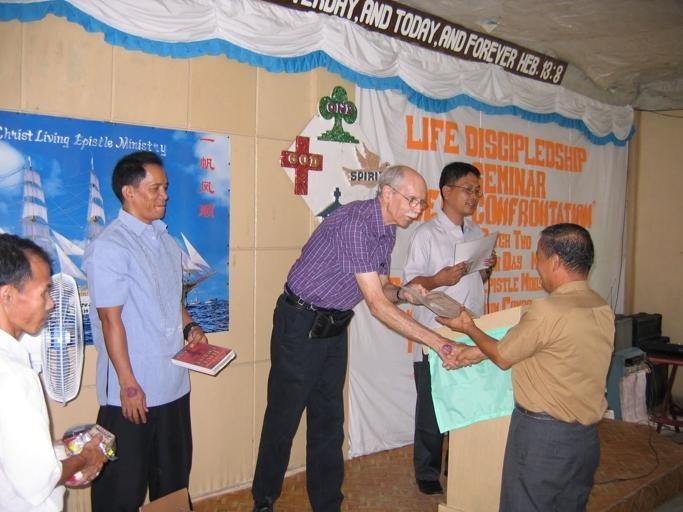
[514, 401, 550, 420]
[279, 283, 322, 313]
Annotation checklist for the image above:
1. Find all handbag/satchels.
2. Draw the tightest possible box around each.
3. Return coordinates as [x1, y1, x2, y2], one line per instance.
[309, 310, 354, 339]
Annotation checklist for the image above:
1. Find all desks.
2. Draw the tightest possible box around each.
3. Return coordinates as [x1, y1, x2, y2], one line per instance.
[644, 354, 683, 433]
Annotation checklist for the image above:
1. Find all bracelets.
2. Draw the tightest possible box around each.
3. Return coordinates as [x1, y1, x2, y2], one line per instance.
[396, 287, 404, 302]
[184, 322, 199, 342]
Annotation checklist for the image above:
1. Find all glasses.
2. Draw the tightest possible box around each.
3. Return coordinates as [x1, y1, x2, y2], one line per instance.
[384, 183, 428, 209]
[445, 184, 481, 196]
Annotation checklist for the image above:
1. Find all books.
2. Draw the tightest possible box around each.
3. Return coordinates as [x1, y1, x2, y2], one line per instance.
[170, 341, 237, 378]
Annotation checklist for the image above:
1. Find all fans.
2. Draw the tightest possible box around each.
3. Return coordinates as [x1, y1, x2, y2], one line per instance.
[18, 271, 85, 407]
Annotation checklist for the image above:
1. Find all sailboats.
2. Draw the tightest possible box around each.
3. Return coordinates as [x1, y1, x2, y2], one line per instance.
[0, 154, 217, 315]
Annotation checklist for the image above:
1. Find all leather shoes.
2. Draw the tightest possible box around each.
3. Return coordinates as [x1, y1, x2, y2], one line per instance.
[253, 503, 273, 512]
[417, 480, 443, 495]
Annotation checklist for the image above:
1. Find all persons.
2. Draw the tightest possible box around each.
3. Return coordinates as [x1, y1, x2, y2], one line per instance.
[251, 165, 473, 512]
[0, 234, 109, 512]
[89, 151, 208, 512]
[433, 222, 616, 512]
[403, 162, 497, 496]
